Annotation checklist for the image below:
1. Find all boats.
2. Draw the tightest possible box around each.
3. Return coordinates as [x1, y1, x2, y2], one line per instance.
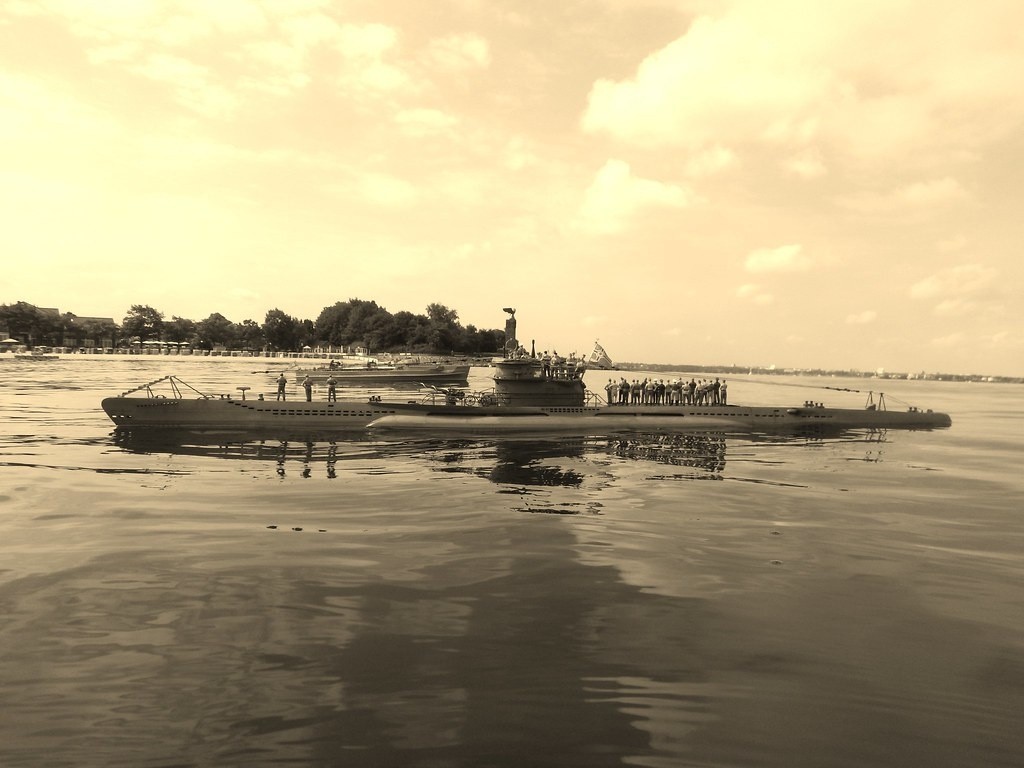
[295, 363, 471, 388]
[101, 340, 952, 431]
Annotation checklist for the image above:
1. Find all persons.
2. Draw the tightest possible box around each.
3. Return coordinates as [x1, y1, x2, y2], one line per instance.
[302, 375, 313, 402]
[542, 350, 586, 380]
[604, 377, 727, 406]
[327, 374, 337, 402]
[276, 373, 287, 401]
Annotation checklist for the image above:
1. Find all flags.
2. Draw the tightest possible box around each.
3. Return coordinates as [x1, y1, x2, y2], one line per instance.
[591, 344, 612, 365]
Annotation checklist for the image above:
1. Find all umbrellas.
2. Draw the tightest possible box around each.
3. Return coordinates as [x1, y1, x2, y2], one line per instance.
[1, 338, 19, 343]
[303, 345, 310, 348]
[132, 340, 190, 345]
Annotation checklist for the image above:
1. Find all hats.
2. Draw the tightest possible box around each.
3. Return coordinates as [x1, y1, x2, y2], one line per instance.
[654, 379, 658, 381]
[673, 379, 677, 382]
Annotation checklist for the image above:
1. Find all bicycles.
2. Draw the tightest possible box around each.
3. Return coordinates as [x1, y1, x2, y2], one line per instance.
[465, 390, 492, 407]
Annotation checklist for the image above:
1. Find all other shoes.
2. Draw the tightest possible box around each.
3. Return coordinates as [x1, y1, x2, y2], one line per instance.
[276, 398, 279, 401]
[283, 399, 285, 401]
[334, 400, 337, 402]
[327, 400, 330, 402]
[608, 402, 728, 407]
[306, 400, 311, 402]
